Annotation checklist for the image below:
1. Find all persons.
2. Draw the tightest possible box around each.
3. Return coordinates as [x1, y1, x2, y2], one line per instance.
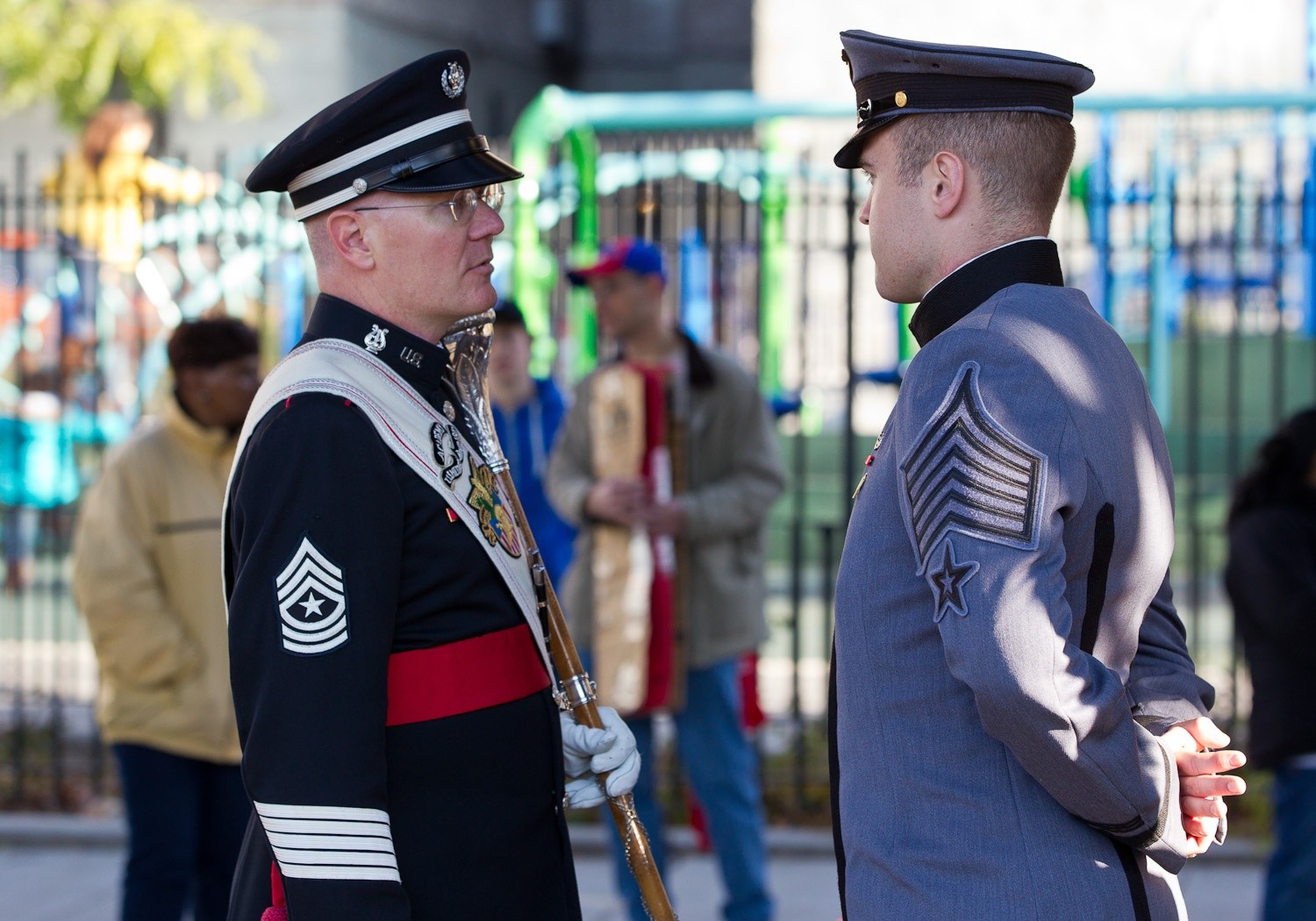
[73, 316, 262, 899]
[40, 101, 217, 399]
[489, 300, 572, 591]
[831, 27, 1244, 921]
[220, 48, 640, 921]
[1224, 408, 1314, 921]
[540, 239, 786, 921]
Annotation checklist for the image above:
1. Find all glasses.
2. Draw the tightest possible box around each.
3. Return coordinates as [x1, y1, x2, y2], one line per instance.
[355, 185, 506, 221]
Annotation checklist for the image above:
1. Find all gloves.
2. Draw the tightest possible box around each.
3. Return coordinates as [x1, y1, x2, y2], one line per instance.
[558, 702, 643, 812]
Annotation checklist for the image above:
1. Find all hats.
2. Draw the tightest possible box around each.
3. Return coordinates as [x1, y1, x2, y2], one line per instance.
[565, 243, 664, 285]
[247, 48, 525, 224]
[834, 30, 1094, 170]
